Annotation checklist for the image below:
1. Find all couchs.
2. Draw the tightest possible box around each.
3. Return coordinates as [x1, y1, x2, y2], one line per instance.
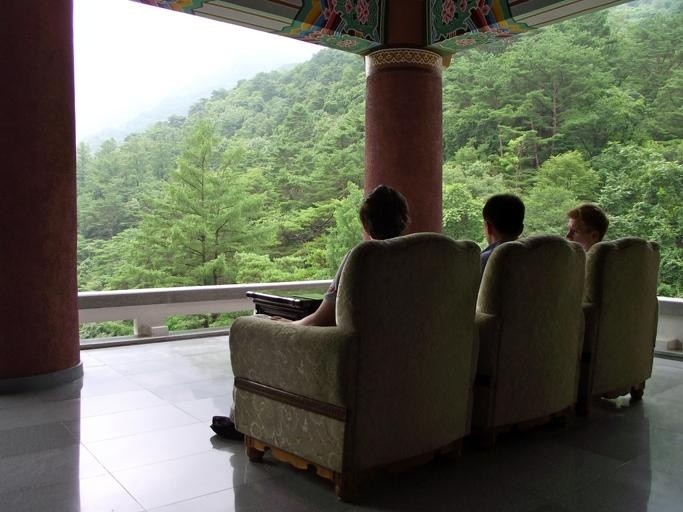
[470, 232, 585, 448]
[230, 234, 482, 495]
[577, 237, 660, 417]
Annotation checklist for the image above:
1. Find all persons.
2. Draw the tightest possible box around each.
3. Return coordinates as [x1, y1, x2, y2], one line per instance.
[565, 204, 608, 253]
[479, 193, 525, 287]
[210, 183, 412, 442]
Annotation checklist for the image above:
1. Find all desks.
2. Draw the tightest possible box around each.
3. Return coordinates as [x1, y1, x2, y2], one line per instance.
[246, 290, 327, 322]
